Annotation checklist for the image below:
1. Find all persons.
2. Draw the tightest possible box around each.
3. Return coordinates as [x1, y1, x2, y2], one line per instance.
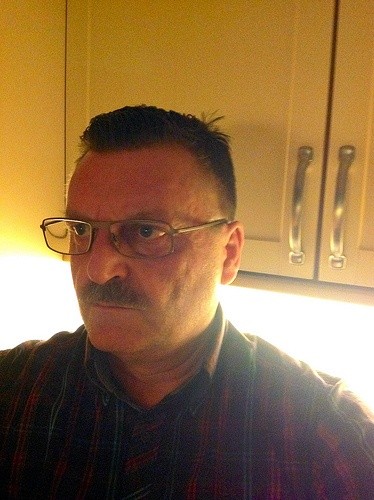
[0, 103, 374, 500]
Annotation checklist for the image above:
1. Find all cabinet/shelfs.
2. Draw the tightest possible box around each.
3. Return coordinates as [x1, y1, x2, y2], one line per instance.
[63, 1, 374, 308]
[0, 0, 67, 256]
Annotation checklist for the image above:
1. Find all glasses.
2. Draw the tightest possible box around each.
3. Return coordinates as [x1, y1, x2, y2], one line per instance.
[39, 216, 230, 261]
